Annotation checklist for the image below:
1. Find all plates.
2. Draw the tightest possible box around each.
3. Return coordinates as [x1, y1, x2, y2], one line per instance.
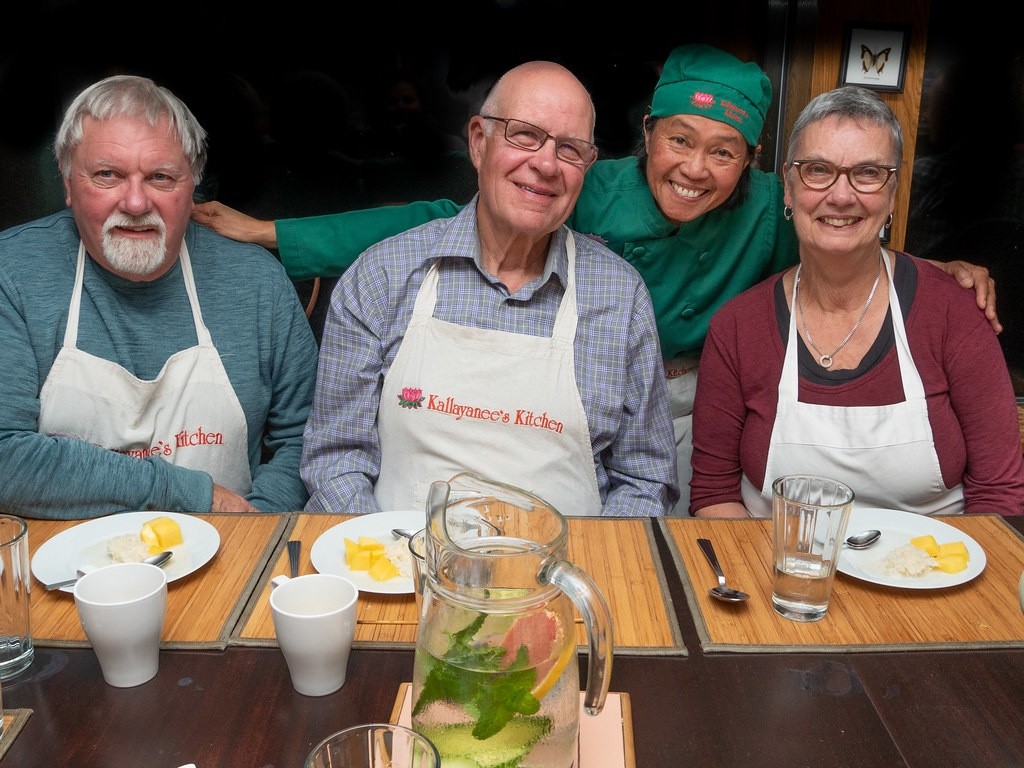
[311, 512, 487, 594]
[798, 508, 987, 589]
[31, 510, 220, 594]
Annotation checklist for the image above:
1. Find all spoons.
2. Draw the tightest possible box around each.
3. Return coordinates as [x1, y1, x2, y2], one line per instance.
[697, 539, 750, 603]
[843, 530, 881, 549]
[391, 528, 421, 540]
[45, 551, 173, 591]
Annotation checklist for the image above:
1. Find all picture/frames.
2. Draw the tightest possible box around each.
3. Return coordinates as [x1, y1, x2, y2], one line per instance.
[837, 18, 913, 94]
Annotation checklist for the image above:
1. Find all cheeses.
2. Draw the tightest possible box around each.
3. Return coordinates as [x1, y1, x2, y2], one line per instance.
[910, 534, 969, 574]
[141, 515, 182, 553]
[344, 536, 399, 580]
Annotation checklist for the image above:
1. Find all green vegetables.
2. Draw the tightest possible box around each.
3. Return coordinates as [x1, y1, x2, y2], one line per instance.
[410, 586, 543, 743]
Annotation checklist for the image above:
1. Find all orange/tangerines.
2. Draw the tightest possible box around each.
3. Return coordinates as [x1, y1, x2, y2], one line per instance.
[496, 610, 574, 698]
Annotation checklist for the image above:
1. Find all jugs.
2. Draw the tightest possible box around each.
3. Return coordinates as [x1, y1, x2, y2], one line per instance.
[410, 471, 611, 768]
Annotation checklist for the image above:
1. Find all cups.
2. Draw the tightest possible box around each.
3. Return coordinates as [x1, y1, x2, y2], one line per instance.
[270, 575, 359, 696]
[0, 516, 35, 683]
[772, 475, 855, 622]
[303, 723, 441, 768]
[408, 517, 499, 627]
[73, 563, 167, 688]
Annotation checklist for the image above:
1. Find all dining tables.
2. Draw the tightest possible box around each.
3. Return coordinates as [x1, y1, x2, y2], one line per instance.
[0, 511, 1024, 768]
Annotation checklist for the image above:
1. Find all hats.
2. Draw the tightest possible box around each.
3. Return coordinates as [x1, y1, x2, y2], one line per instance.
[648, 45, 773, 147]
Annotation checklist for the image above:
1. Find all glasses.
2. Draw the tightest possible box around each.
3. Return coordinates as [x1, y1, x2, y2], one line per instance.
[786, 154, 899, 193]
[484, 116, 599, 165]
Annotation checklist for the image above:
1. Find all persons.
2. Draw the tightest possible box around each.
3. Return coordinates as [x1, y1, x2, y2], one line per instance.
[188, 48, 1002, 523]
[905, 68, 1001, 284]
[0, 75, 320, 522]
[301, 60, 680, 518]
[688, 86, 1024, 519]
[207, 77, 451, 216]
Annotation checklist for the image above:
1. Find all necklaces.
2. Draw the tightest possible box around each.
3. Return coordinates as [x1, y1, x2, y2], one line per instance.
[797, 254, 882, 369]
